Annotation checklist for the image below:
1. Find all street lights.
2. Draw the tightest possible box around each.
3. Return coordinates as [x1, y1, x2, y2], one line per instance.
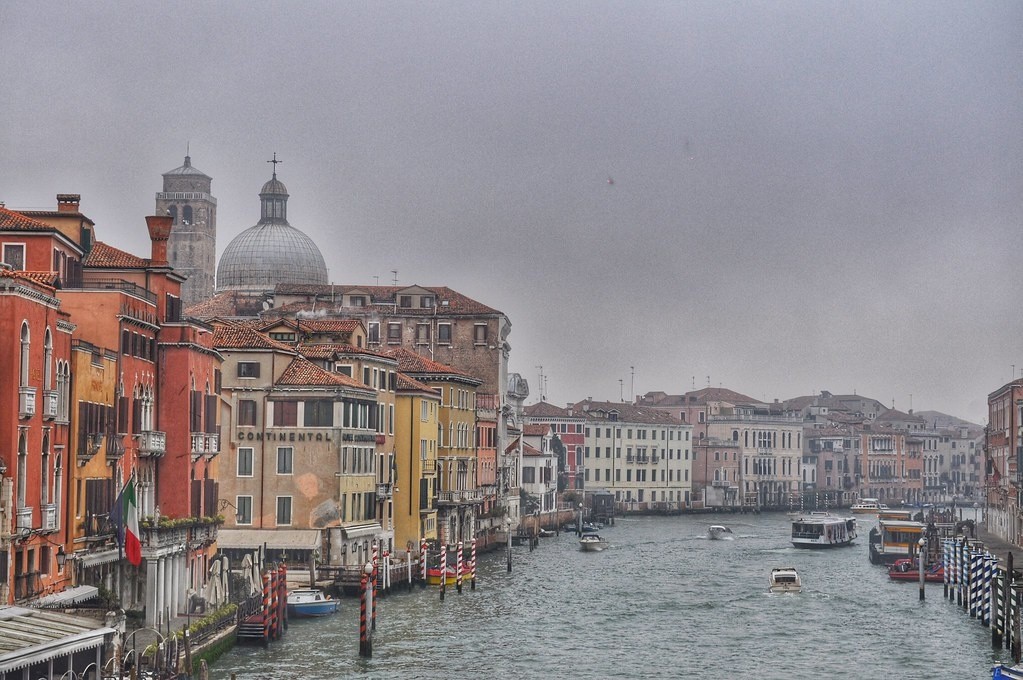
[918, 538, 925, 600]
[364, 561, 376, 658]
[579, 502, 582, 538]
[507, 518, 512, 573]
[973, 503, 979, 539]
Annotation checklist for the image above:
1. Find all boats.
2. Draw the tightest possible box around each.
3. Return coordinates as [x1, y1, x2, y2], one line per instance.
[287, 590, 342, 617]
[790, 512, 858, 549]
[768, 567, 802, 593]
[579, 534, 610, 550]
[425, 564, 475, 585]
[870, 511, 923, 566]
[888, 556, 971, 582]
[851, 497, 890, 514]
[707, 525, 733, 538]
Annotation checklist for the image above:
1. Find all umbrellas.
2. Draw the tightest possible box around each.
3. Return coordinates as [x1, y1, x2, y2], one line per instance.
[252, 550, 264, 597]
[241, 554, 255, 596]
[205, 556, 230, 614]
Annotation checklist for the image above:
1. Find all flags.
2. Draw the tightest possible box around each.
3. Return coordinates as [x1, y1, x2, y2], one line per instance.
[109, 472, 142, 565]
